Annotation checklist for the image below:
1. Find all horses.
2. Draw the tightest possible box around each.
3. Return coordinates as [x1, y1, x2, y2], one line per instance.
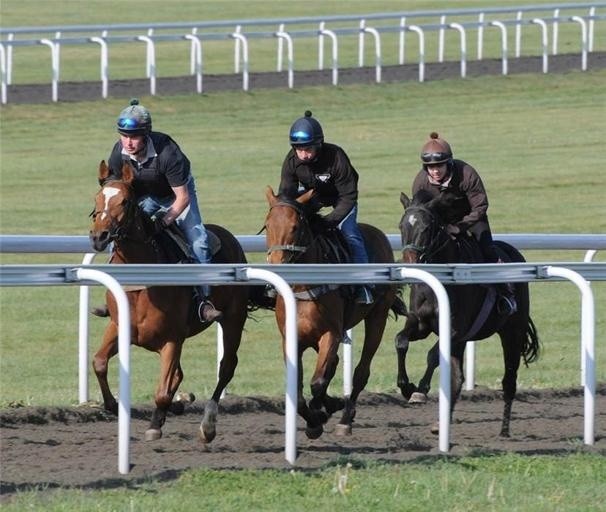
[88, 159, 278, 444]
[263, 184, 409, 439]
[394, 189, 540, 438]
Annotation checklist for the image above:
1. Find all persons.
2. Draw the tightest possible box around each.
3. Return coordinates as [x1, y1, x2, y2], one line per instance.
[276, 111, 376, 306]
[91, 99, 223, 326]
[412, 132, 499, 263]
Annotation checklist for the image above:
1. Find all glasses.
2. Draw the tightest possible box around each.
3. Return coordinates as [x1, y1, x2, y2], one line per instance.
[289, 131, 323, 141]
[118, 118, 150, 128]
[422, 152, 452, 162]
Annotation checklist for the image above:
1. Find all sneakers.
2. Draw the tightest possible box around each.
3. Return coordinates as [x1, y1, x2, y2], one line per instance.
[500, 295, 520, 311]
[355, 288, 375, 301]
[91, 304, 109, 316]
[193, 297, 224, 322]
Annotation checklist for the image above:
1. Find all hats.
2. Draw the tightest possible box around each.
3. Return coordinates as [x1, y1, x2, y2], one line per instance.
[117, 100, 151, 136]
[422, 132, 452, 166]
[290, 111, 324, 146]
